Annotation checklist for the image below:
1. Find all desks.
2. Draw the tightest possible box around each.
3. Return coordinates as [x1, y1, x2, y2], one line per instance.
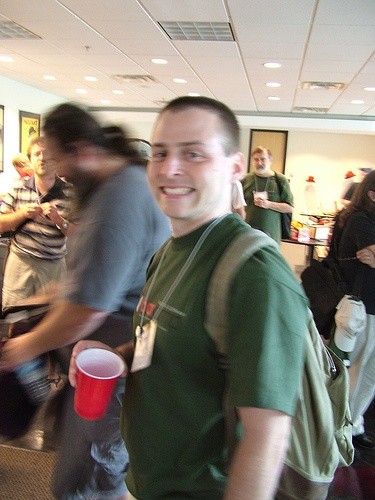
[300, 213, 336, 223]
[282, 237, 331, 266]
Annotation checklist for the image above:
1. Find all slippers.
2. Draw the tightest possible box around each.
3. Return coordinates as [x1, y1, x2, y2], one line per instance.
[48, 375, 64, 392]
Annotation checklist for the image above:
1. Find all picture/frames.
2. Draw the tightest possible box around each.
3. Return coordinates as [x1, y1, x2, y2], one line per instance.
[0, 104, 4, 172]
[19, 110, 40, 162]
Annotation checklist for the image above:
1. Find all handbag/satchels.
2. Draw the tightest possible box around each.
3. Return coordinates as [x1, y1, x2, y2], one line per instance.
[275, 171, 292, 239]
[300, 255, 348, 340]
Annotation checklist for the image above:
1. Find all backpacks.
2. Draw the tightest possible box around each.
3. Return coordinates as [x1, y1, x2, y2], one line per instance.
[203, 228, 356, 500]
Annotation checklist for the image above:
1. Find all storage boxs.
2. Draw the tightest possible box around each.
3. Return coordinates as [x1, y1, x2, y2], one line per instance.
[290, 220, 310, 242]
[305, 225, 329, 239]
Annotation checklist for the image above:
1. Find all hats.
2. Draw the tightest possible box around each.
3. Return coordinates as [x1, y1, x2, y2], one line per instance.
[128, 141, 152, 161]
[335, 294, 366, 353]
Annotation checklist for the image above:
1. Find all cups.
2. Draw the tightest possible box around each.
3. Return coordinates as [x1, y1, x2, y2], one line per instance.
[74, 348, 126, 420]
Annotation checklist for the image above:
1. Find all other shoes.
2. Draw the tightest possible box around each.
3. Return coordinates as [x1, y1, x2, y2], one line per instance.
[354, 432, 375, 449]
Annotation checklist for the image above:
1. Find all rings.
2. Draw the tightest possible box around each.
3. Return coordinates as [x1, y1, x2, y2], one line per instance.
[367, 255, 370, 260]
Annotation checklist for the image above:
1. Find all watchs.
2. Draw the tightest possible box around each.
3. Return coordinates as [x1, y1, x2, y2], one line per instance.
[55, 219, 68, 230]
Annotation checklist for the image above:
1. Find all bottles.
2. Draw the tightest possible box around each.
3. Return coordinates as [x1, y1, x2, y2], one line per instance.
[11, 358, 55, 404]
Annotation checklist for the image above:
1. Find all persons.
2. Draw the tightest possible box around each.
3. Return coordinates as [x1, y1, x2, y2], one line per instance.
[328, 167, 375, 450]
[0, 102, 173, 500]
[231, 145, 295, 249]
[0, 137, 74, 394]
[68, 96, 310, 500]
